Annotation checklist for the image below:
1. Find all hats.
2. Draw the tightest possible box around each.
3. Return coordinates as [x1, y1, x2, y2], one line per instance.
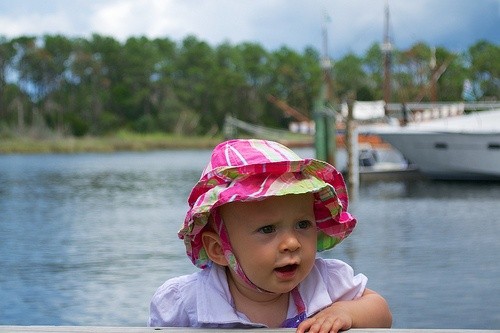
[180, 139, 358, 268]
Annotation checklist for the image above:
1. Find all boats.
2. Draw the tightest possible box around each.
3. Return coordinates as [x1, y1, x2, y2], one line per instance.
[357, 107, 499, 183]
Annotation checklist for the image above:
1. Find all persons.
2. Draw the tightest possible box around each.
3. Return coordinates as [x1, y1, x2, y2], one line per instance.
[147, 137, 393, 332]
